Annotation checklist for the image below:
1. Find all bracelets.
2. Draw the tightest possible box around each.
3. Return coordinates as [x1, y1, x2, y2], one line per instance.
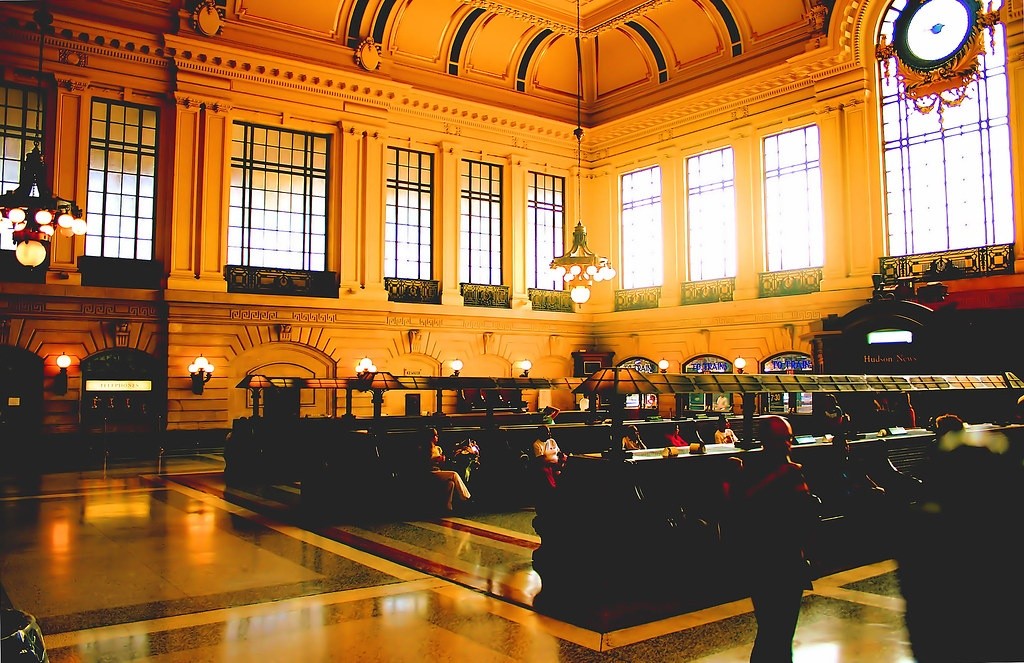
[638, 440, 642, 443]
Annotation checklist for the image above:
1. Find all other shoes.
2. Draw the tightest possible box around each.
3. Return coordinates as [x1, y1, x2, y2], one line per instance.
[463, 497, 481, 506]
[446, 508, 464, 517]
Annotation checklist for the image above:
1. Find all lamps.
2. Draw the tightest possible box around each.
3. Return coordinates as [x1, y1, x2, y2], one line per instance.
[451, 359, 463, 377]
[519, 360, 532, 377]
[54, 352, 71, 396]
[354, 372, 404, 419]
[355, 356, 377, 378]
[734, 356, 746, 374]
[658, 358, 669, 373]
[188, 354, 214, 396]
[570, 368, 661, 460]
[548, 0, 615, 311]
[234, 374, 276, 419]
[0, 7, 90, 273]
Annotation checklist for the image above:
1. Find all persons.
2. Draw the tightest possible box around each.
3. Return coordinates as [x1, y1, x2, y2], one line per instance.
[533, 426, 568, 476]
[544, 407, 560, 424]
[716, 394, 728, 411]
[646, 395, 656, 408]
[426, 429, 471, 511]
[715, 419, 737, 444]
[622, 425, 647, 450]
[665, 424, 688, 446]
[727, 392, 1023, 662]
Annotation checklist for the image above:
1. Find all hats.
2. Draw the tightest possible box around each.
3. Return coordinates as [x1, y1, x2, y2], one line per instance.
[758, 416, 799, 445]
